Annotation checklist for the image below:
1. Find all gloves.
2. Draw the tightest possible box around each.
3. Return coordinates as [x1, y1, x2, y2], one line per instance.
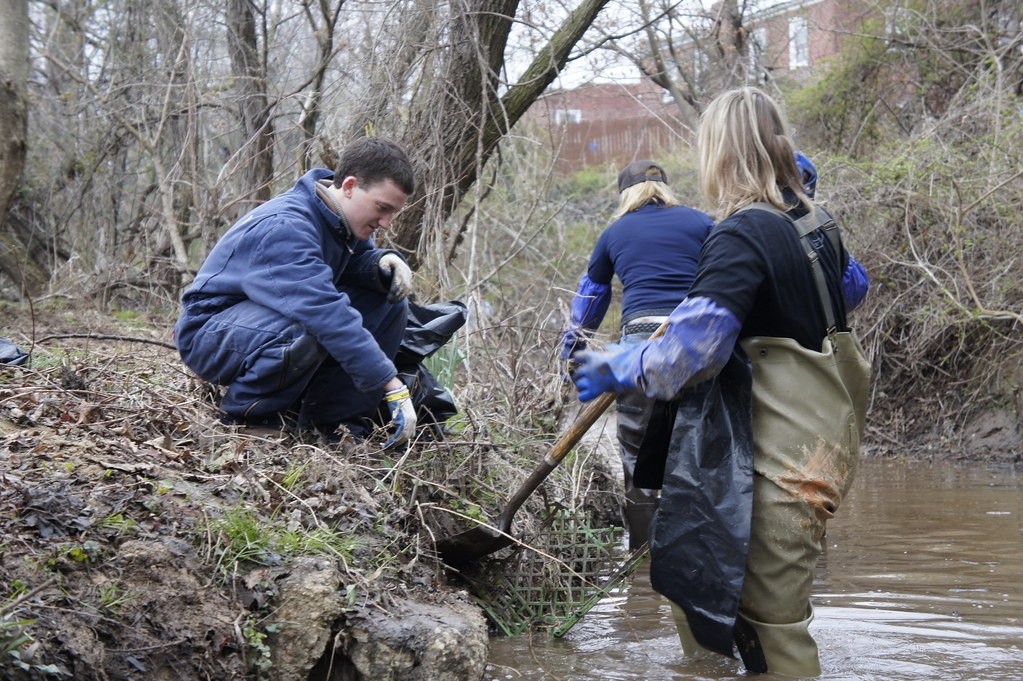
[378, 252, 413, 304]
[558, 357, 574, 386]
[570, 342, 651, 402]
[379, 384, 418, 451]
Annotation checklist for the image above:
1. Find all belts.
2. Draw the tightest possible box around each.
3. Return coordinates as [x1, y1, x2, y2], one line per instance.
[619, 323, 663, 335]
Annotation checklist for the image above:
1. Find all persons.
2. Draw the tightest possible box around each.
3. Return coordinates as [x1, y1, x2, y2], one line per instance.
[174, 137, 417, 450]
[560, 160, 715, 555]
[573, 86, 872, 677]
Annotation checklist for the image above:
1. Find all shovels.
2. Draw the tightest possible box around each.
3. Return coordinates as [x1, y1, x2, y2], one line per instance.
[429, 318, 669, 565]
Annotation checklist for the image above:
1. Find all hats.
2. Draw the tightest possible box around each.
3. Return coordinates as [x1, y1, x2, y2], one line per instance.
[617, 159, 668, 193]
[780, 150, 818, 203]
